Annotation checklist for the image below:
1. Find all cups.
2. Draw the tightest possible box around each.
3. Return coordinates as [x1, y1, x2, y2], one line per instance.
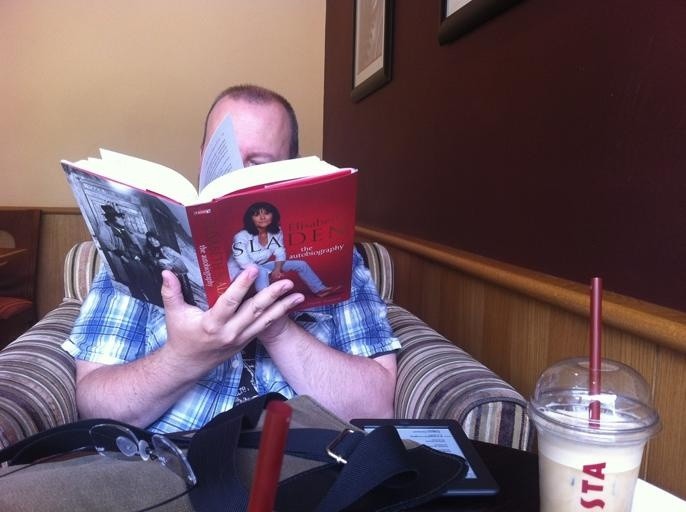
[527, 359, 659, 512]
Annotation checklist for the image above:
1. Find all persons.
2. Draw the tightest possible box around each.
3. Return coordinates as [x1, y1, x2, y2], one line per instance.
[228, 201, 342, 299]
[96, 204, 150, 289]
[143, 232, 189, 302]
[63, 85, 401, 437]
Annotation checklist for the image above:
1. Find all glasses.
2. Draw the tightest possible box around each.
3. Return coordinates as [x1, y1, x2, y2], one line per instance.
[0, 424, 197, 512]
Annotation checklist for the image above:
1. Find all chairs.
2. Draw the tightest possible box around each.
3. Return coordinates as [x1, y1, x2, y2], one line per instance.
[3, 235, 534, 454]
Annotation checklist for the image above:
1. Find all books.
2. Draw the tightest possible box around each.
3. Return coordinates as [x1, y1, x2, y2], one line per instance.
[57, 147, 357, 311]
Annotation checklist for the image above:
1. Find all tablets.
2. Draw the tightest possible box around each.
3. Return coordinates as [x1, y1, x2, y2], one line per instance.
[351, 419, 500, 497]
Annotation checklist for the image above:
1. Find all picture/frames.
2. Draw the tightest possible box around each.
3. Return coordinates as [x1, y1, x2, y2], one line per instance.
[348, 0, 396, 103]
[435, 0, 511, 46]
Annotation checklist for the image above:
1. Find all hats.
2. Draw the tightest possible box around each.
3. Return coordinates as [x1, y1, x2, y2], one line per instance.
[187, 393, 467, 512]
